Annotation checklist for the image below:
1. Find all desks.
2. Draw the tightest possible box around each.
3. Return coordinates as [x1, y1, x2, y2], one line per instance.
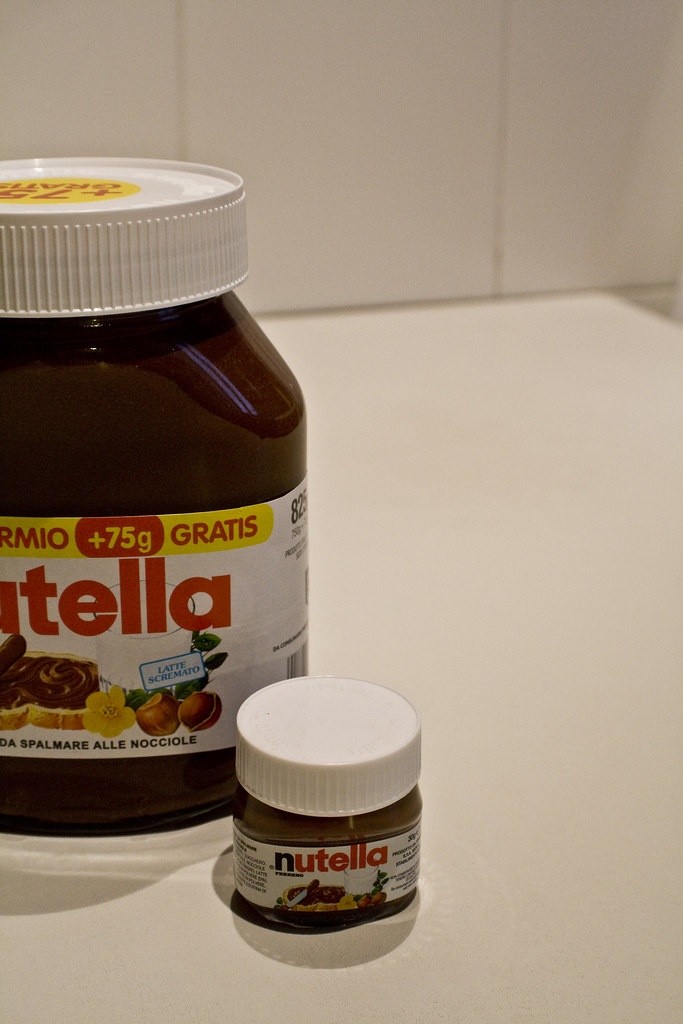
[4, 287, 681, 1021]
